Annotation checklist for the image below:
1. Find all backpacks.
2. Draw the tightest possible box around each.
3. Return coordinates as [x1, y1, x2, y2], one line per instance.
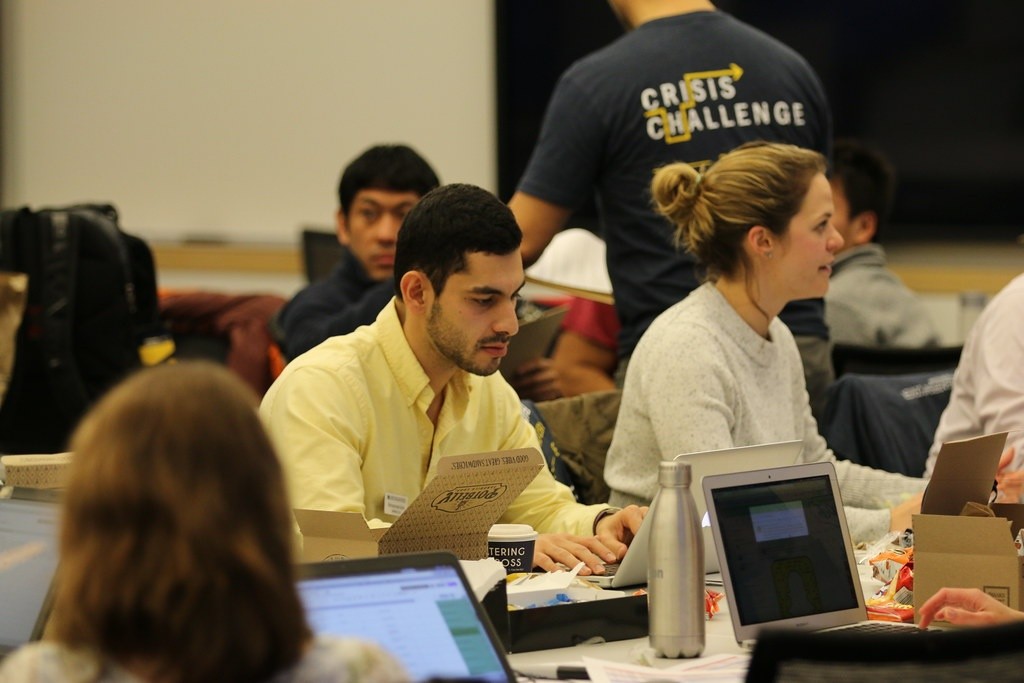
[0, 206, 159, 456]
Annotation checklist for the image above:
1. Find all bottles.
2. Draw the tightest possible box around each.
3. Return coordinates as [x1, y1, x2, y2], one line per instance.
[648, 462, 705, 659]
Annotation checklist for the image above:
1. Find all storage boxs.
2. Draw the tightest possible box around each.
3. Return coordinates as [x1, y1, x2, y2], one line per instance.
[912, 431, 1024, 625]
[294, 448, 545, 563]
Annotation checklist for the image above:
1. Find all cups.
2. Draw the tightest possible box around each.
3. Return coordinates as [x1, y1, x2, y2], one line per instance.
[488, 525, 537, 576]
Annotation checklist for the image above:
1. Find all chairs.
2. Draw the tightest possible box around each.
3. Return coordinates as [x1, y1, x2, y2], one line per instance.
[746, 341, 1023, 683]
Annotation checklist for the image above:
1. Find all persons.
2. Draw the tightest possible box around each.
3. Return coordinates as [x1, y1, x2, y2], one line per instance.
[919, 587, 1024, 630]
[923, 273, 1024, 504]
[0, 359, 413, 683]
[273, 145, 559, 403]
[259, 182, 651, 575]
[506, 0, 829, 388]
[552, 297, 620, 397]
[603, 140, 1024, 545]
[823, 146, 940, 348]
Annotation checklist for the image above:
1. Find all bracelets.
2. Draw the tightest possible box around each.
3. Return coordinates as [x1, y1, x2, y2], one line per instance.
[593, 508, 617, 536]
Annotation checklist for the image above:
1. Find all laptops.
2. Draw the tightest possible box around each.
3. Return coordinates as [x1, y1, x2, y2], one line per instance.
[295, 550, 517, 683]
[533, 439, 805, 589]
[1, 484, 67, 665]
[701, 462, 947, 650]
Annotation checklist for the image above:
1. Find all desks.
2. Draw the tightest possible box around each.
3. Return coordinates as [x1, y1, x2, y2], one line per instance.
[505, 584, 754, 682]
